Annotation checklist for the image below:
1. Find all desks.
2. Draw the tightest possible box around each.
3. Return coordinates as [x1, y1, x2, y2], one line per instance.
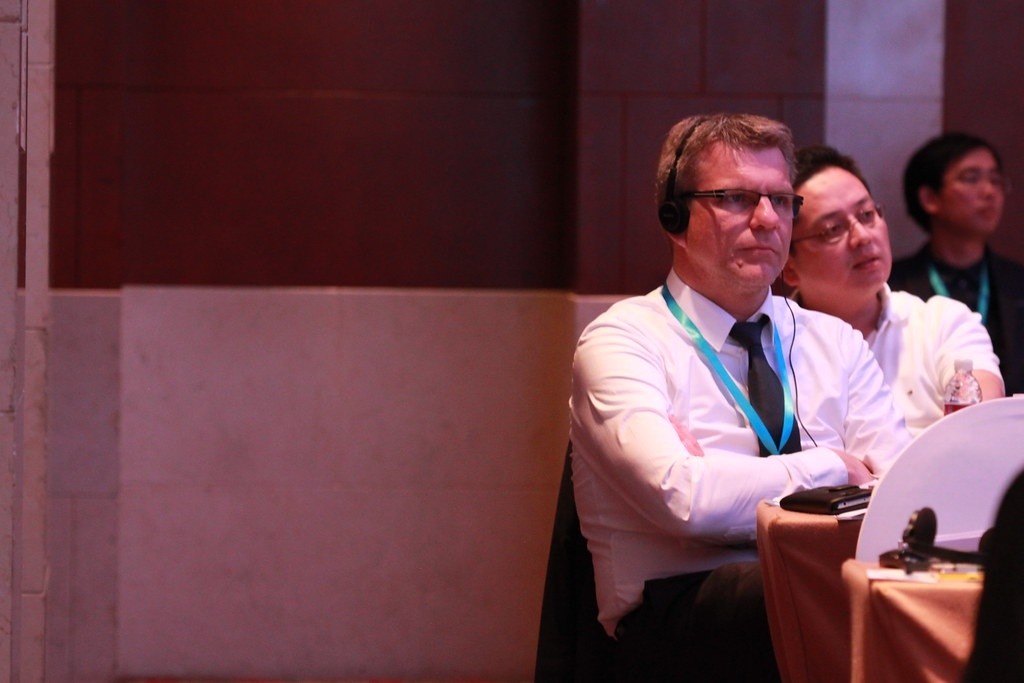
[755, 492, 987, 683]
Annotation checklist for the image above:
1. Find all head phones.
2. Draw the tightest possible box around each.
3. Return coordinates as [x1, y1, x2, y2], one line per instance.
[657, 118, 708, 236]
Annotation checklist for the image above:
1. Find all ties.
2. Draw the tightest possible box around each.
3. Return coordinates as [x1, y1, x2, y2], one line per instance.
[729, 314, 802, 457]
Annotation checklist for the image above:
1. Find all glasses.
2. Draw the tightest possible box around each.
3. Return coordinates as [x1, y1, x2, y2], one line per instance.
[682, 188, 803, 218]
[793, 203, 885, 244]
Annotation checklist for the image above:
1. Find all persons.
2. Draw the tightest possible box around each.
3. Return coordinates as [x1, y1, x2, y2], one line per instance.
[566, 113, 917, 683]
[889, 133, 1024, 395]
[778, 142, 1005, 435]
